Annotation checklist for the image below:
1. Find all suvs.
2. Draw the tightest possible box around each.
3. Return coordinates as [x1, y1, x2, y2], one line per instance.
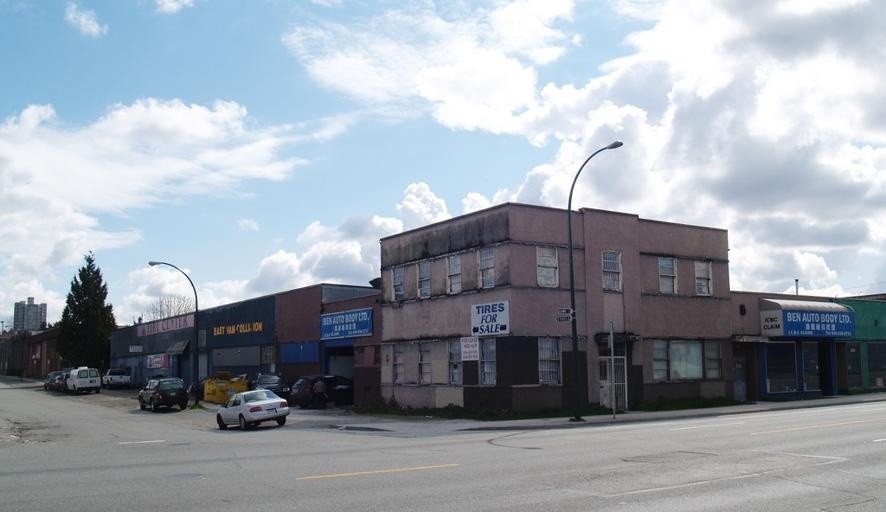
[250, 372, 290, 397]
[289, 374, 353, 408]
[137, 377, 190, 413]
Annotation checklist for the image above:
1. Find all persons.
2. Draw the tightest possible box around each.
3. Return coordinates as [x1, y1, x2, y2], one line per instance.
[313, 377, 329, 410]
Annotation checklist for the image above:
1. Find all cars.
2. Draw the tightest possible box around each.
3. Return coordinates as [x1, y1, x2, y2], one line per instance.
[214, 389, 290, 431]
[44, 370, 68, 392]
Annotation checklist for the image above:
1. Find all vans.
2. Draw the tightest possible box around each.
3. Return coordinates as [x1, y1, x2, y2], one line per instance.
[66, 366, 102, 394]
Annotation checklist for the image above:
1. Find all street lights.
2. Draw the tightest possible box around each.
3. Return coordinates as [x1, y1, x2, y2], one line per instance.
[564, 138, 626, 422]
[4, 324, 17, 332]
[146, 260, 200, 407]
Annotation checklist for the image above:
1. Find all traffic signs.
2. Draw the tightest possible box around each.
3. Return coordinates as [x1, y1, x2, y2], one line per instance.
[557, 308, 573, 314]
[557, 315, 572, 324]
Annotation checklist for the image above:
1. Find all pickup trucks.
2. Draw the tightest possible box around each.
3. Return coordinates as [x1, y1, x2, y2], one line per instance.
[101, 368, 132, 387]
